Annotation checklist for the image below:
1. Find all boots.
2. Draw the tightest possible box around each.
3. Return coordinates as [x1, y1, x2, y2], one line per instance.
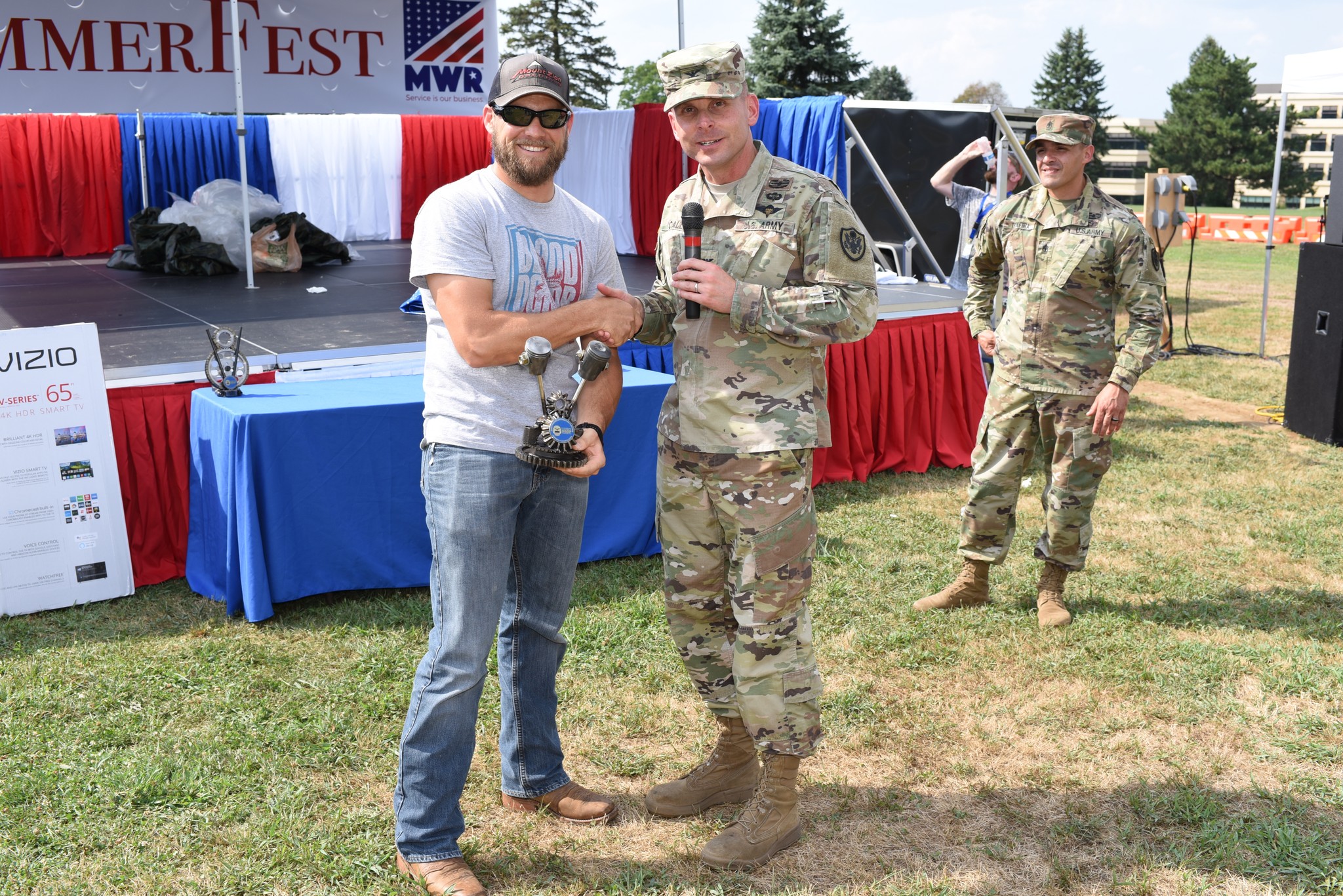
[1035, 561, 1073, 630]
[697, 753, 802, 871]
[913, 561, 994, 612]
[643, 717, 758, 820]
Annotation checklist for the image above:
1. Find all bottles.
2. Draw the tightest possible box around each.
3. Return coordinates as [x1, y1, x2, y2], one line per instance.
[977, 136, 996, 170]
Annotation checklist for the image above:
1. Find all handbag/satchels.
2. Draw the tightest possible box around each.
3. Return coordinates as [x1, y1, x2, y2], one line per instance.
[251, 222, 303, 274]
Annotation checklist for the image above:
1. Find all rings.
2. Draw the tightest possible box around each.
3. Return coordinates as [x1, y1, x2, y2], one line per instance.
[695, 282, 699, 293]
[1111, 418, 1119, 421]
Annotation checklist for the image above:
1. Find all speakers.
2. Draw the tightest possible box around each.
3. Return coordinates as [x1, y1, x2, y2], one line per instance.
[1282, 135, 1343, 447]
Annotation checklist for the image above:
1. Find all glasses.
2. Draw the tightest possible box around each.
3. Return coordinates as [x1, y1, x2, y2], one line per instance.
[490, 103, 571, 130]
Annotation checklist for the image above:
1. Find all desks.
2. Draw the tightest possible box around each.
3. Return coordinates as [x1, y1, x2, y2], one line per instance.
[184, 361, 675, 625]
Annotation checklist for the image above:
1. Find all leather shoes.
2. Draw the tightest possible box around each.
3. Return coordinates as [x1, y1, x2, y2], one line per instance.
[395, 850, 489, 896]
[499, 782, 619, 825]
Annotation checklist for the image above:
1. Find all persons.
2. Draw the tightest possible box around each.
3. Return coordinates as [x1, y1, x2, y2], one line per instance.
[595, 40, 878, 871]
[913, 110, 1167, 629]
[393, 54, 636, 896]
[930, 138, 1024, 294]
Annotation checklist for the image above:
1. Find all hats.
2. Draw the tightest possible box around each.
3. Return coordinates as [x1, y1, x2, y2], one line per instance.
[1007, 150, 1025, 188]
[655, 41, 746, 113]
[488, 53, 574, 114]
[1025, 113, 1096, 151]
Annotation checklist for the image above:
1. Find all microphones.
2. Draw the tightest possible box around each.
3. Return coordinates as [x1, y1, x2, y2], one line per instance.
[681, 201, 705, 319]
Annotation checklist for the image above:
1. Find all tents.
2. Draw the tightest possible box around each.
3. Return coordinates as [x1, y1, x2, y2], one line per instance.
[0, 1, 687, 290]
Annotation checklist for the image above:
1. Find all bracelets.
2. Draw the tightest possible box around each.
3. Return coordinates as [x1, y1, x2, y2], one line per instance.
[577, 423, 605, 447]
